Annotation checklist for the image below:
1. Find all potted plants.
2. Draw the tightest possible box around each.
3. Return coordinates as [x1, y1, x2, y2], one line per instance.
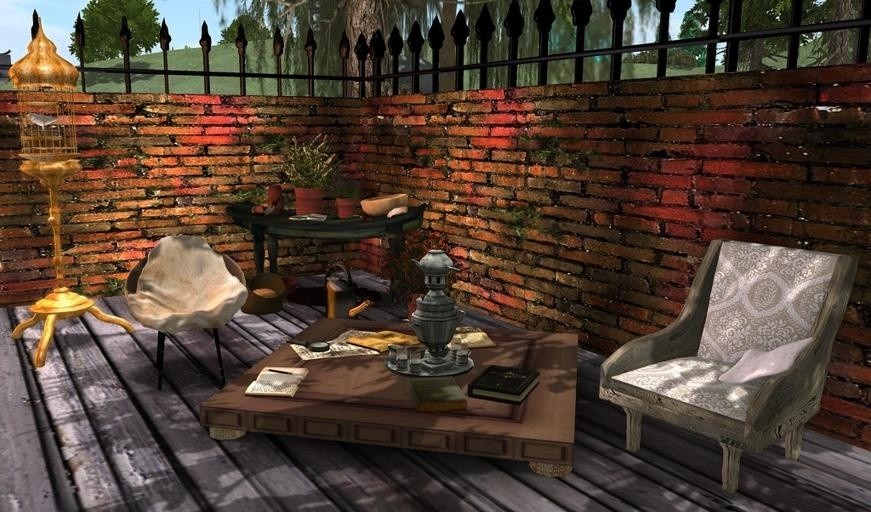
[257, 135, 362, 218]
[381, 232, 463, 318]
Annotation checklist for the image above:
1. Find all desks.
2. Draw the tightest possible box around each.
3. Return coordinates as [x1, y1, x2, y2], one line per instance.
[226, 198, 425, 307]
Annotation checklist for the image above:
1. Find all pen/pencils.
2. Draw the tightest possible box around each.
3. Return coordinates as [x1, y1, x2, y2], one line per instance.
[269, 369, 293, 374]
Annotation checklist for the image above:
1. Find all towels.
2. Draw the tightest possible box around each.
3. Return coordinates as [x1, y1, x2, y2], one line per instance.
[717, 338, 813, 386]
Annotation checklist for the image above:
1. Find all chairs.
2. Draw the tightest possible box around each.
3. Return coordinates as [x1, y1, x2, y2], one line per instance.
[126, 243, 249, 391]
[597, 239, 863, 499]
